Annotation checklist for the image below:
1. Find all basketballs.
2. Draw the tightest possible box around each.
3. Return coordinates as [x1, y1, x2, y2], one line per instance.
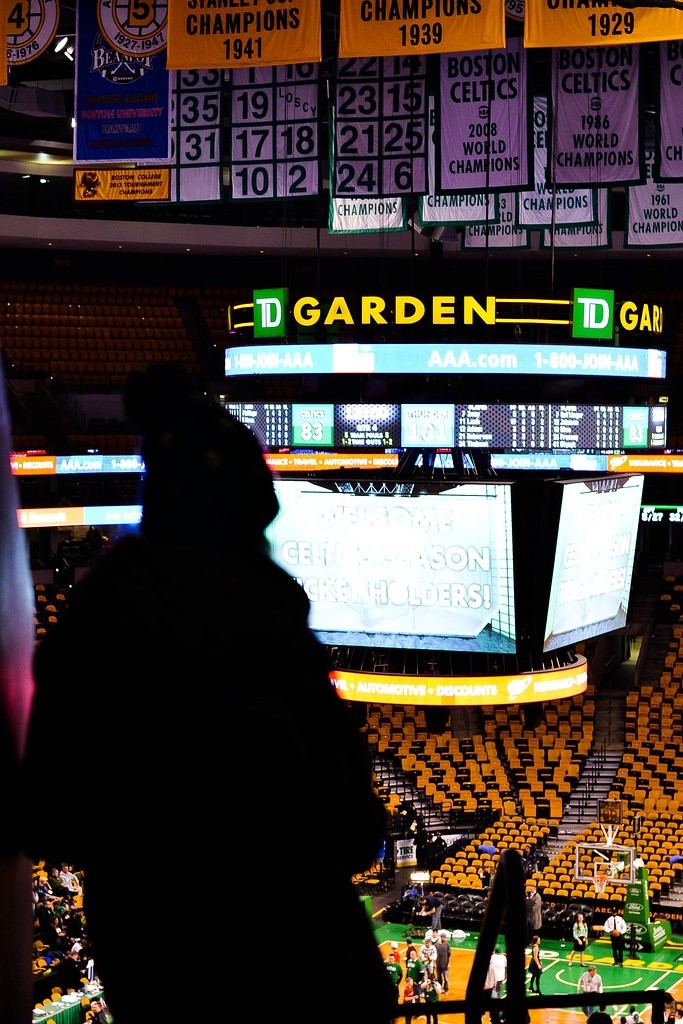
[611, 930, 620, 937]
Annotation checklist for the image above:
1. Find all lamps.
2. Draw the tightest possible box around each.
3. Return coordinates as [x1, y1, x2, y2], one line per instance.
[51, 35, 69, 53]
[64, 39, 75, 62]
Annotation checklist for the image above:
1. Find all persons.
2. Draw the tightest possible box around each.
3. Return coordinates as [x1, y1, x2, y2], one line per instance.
[522, 844, 548, 880]
[662, 992, 683, 1024]
[480, 866, 491, 889]
[604, 907, 628, 968]
[433, 831, 447, 866]
[578, 965, 643, 1024]
[525, 887, 543, 939]
[17, 397, 395, 1024]
[478, 945, 508, 1023]
[569, 913, 588, 967]
[382, 929, 451, 1024]
[31, 863, 110, 1024]
[528, 935, 542, 993]
[542, 904, 587, 941]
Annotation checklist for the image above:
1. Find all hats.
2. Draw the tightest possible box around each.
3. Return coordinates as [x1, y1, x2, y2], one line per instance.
[123, 359, 281, 548]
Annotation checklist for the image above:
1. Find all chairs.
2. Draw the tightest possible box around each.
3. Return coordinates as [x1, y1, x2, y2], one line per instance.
[0, 272, 683, 1024]
